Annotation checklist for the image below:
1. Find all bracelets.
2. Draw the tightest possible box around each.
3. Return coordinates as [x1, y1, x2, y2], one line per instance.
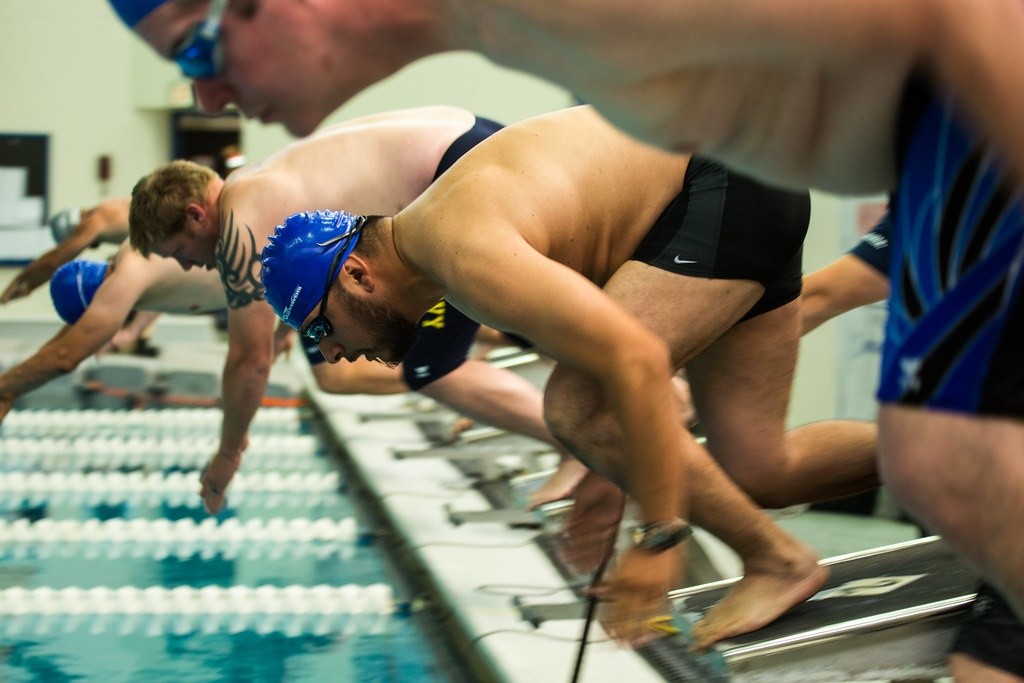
[630, 518, 693, 554]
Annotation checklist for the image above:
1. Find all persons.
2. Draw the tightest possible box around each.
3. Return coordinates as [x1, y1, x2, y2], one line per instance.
[0, 0, 1024, 683]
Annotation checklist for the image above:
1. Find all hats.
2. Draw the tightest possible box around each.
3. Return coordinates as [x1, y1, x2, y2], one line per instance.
[257, 209, 365, 329]
[51, 209, 80, 245]
[108, 1, 167, 28]
[50, 260, 109, 324]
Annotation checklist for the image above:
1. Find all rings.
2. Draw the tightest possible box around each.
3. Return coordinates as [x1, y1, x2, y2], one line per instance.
[212, 489, 220, 495]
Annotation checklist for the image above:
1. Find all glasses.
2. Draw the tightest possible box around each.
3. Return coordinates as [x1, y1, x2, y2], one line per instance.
[175, 0, 227, 80]
[299, 214, 367, 345]
